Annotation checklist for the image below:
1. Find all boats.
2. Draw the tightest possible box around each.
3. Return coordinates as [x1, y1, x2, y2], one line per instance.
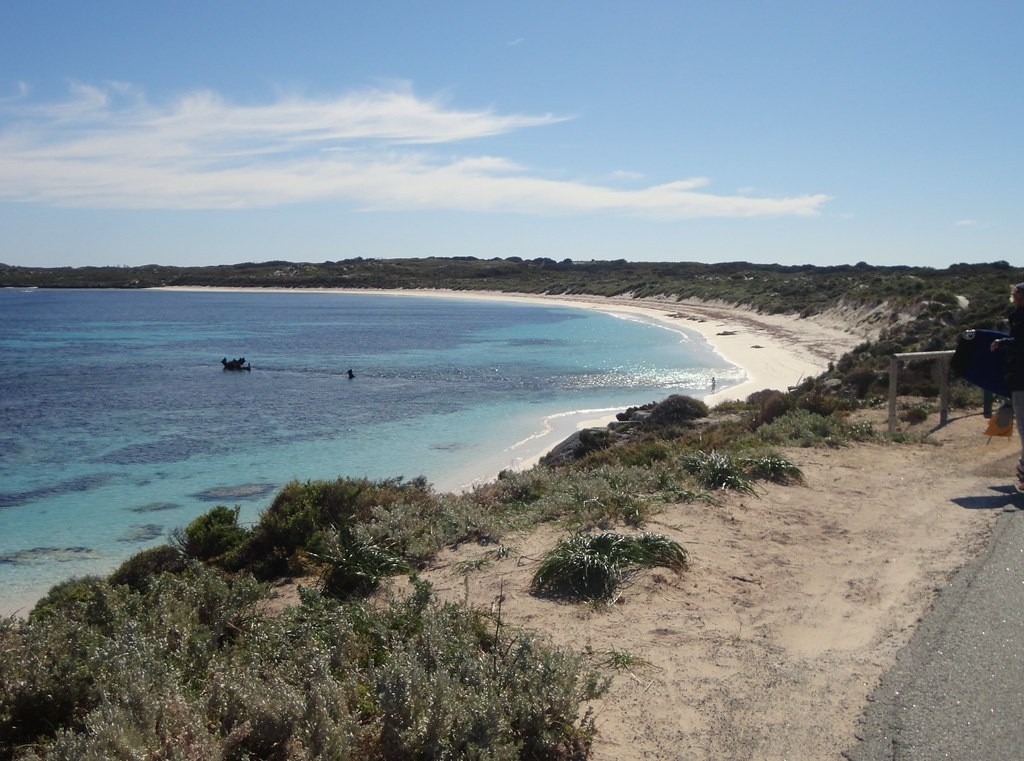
[221, 358, 251, 371]
[348, 369, 355, 379]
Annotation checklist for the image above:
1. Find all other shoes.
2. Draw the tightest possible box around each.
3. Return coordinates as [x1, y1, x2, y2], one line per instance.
[1015, 482, 1024, 494]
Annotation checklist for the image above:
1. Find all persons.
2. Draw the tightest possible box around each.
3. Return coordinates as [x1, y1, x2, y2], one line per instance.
[989, 282, 1024, 493]
